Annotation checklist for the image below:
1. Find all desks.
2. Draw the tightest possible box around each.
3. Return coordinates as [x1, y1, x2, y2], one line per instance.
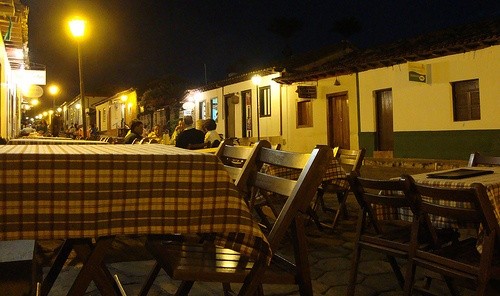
[387, 166, 500, 240]
[0, 136, 216, 296]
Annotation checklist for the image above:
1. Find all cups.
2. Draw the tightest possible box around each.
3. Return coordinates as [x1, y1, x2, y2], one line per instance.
[163, 133, 169, 144]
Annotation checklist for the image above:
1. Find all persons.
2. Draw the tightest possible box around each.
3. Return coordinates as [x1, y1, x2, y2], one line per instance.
[21, 116, 60, 137]
[123, 116, 221, 150]
[65, 123, 96, 140]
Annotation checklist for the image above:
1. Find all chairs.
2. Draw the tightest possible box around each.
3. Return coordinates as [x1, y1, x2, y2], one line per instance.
[117, 139, 500, 296]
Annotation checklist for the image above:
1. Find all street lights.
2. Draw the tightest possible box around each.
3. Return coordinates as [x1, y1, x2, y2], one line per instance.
[71, 18, 89, 140]
[252, 74, 260, 141]
[49, 85, 56, 137]
